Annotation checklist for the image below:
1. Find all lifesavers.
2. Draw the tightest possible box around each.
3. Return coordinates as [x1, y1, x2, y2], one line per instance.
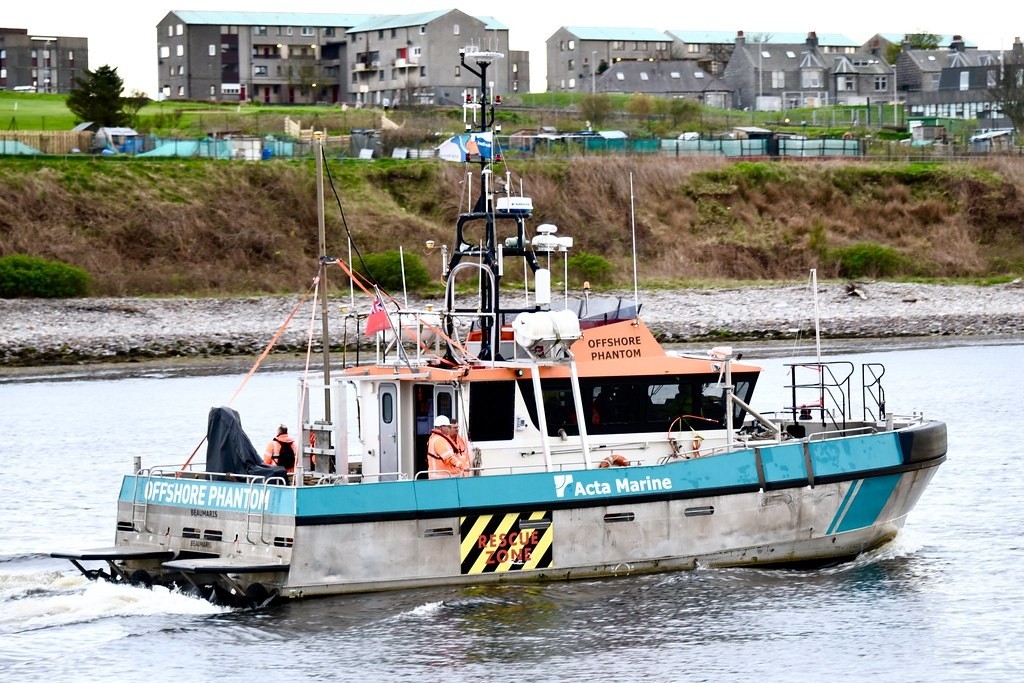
[598, 455, 630, 468]
[840, 131, 854, 140]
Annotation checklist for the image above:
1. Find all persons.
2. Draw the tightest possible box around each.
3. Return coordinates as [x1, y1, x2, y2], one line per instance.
[426, 415, 470, 481]
[262, 425, 301, 475]
[307, 424, 318, 471]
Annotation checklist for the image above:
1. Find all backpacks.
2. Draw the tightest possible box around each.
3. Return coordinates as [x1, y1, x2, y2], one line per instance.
[271, 438, 297, 468]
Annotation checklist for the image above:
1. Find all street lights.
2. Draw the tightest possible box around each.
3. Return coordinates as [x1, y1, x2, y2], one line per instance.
[250, 63, 254, 103]
[891, 65, 897, 127]
[759, 40, 765, 111]
[592, 51, 597, 92]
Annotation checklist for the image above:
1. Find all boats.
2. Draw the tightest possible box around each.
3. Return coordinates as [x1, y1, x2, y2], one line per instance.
[49, 36, 948, 608]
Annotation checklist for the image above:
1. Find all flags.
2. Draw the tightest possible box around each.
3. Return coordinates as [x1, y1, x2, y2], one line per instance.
[362, 289, 394, 337]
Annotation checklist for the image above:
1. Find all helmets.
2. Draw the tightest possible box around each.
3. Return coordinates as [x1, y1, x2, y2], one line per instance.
[434, 415, 452, 427]
